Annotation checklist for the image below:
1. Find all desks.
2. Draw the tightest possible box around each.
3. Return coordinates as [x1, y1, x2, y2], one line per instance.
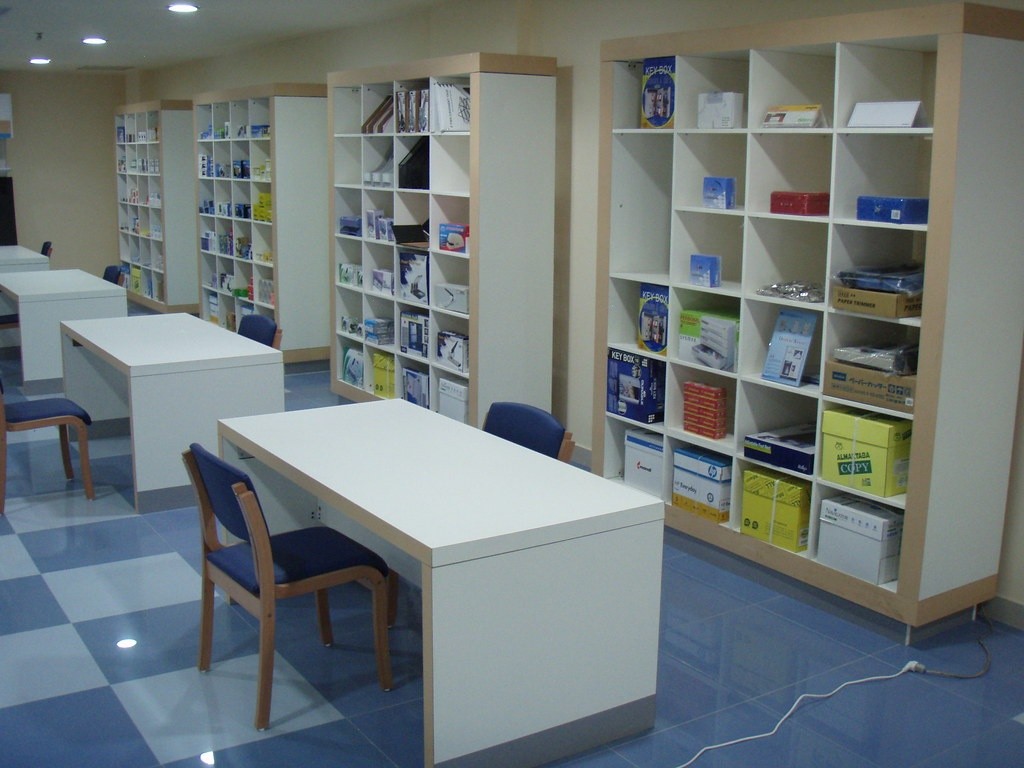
[61, 313, 283, 517]
[219, 399, 667, 767]
[0, 245, 49, 316]
[0, 268, 128, 382]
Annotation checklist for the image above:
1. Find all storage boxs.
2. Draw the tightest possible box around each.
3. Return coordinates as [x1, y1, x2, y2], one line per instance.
[253, 192, 272, 222]
[818, 496, 902, 586]
[198, 124, 212, 139]
[199, 199, 215, 215]
[216, 201, 230, 217]
[199, 153, 213, 177]
[251, 124, 273, 138]
[624, 426, 664, 497]
[237, 125, 249, 140]
[214, 121, 230, 139]
[338, 210, 470, 425]
[234, 159, 250, 180]
[117, 125, 164, 301]
[215, 163, 231, 178]
[703, 177, 737, 209]
[856, 195, 929, 224]
[235, 203, 251, 218]
[823, 360, 916, 412]
[673, 447, 731, 524]
[690, 254, 722, 287]
[770, 191, 829, 217]
[832, 284, 923, 318]
[741, 469, 809, 552]
[201, 231, 276, 327]
[821, 407, 911, 498]
[698, 92, 744, 129]
[608, 349, 665, 424]
[684, 382, 726, 439]
[744, 421, 816, 474]
[677, 308, 741, 373]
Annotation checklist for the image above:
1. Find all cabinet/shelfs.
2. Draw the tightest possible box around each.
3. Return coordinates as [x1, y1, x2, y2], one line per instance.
[113, 98, 200, 314]
[325, 50, 558, 432]
[190, 83, 330, 364]
[593, 0, 1024, 647]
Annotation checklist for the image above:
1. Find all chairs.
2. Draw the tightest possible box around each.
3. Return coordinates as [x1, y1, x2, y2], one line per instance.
[182, 443, 396, 730]
[40, 239, 53, 256]
[482, 401, 576, 461]
[103, 264, 125, 284]
[0, 383, 95, 514]
[237, 312, 284, 348]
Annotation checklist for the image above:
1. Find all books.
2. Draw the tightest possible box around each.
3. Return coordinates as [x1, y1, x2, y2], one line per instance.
[395, 88, 429, 133]
